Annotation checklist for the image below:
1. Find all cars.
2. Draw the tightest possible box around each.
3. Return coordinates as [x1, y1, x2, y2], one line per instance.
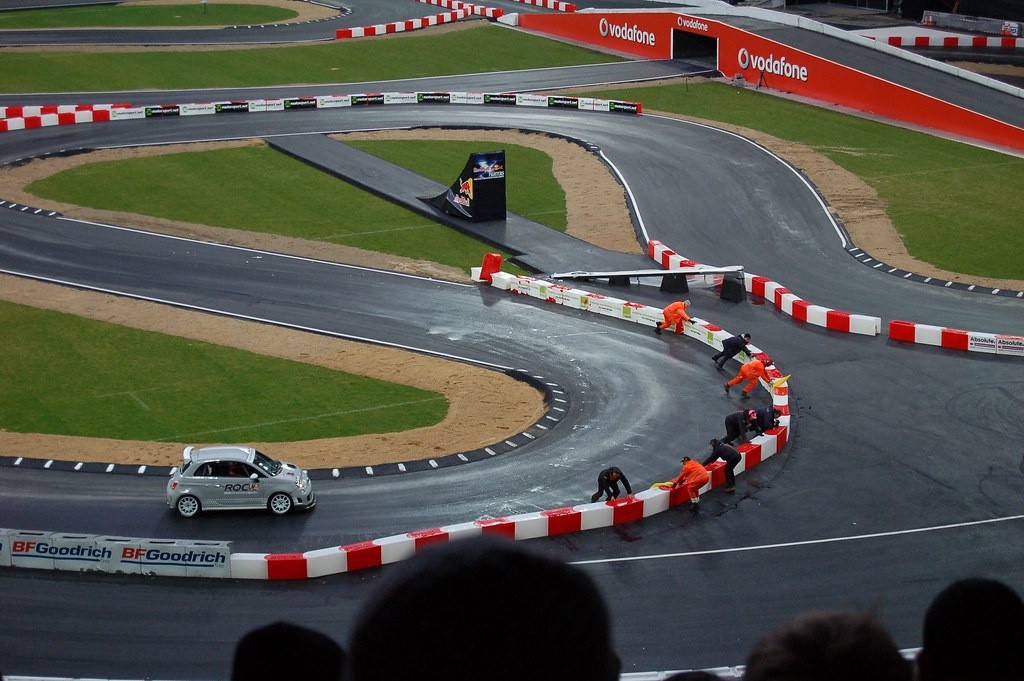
[166, 445, 317, 518]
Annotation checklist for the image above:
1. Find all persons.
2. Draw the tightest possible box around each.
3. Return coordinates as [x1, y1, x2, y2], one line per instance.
[230, 621, 346, 681]
[662, 613, 911, 681]
[712, 333, 756, 371]
[654, 300, 697, 335]
[915, 577, 1024, 681]
[671, 456, 709, 511]
[229, 464, 241, 477]
[749, 406, 782, 433]
[724, 359, 773, 399]
[347, 534, 624, 681]
[702, 439, 741, 492]
[591, 466, 634, 503]
[721, 410, 764, 446]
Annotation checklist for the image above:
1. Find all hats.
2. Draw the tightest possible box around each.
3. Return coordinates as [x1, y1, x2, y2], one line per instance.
[766, 359, 772, 366]
[708, 439, 718, 444]
[679, 456, 691, 463]
[608, 466, 622, 476]
[684, 300, 691, 306]
[749, 409, 757, 419]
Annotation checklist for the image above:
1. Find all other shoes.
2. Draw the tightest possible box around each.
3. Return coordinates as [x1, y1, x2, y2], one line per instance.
[717, 365, 725, 370]
[690, 502, 700, 510]
[726, 487, 735, 492]
[655, 327, 661, 335]
[656, 322, 661, 327]
[742, 391, 751, 398]
[724, 383, 730, 394]
[712, 356, 719, 364]
